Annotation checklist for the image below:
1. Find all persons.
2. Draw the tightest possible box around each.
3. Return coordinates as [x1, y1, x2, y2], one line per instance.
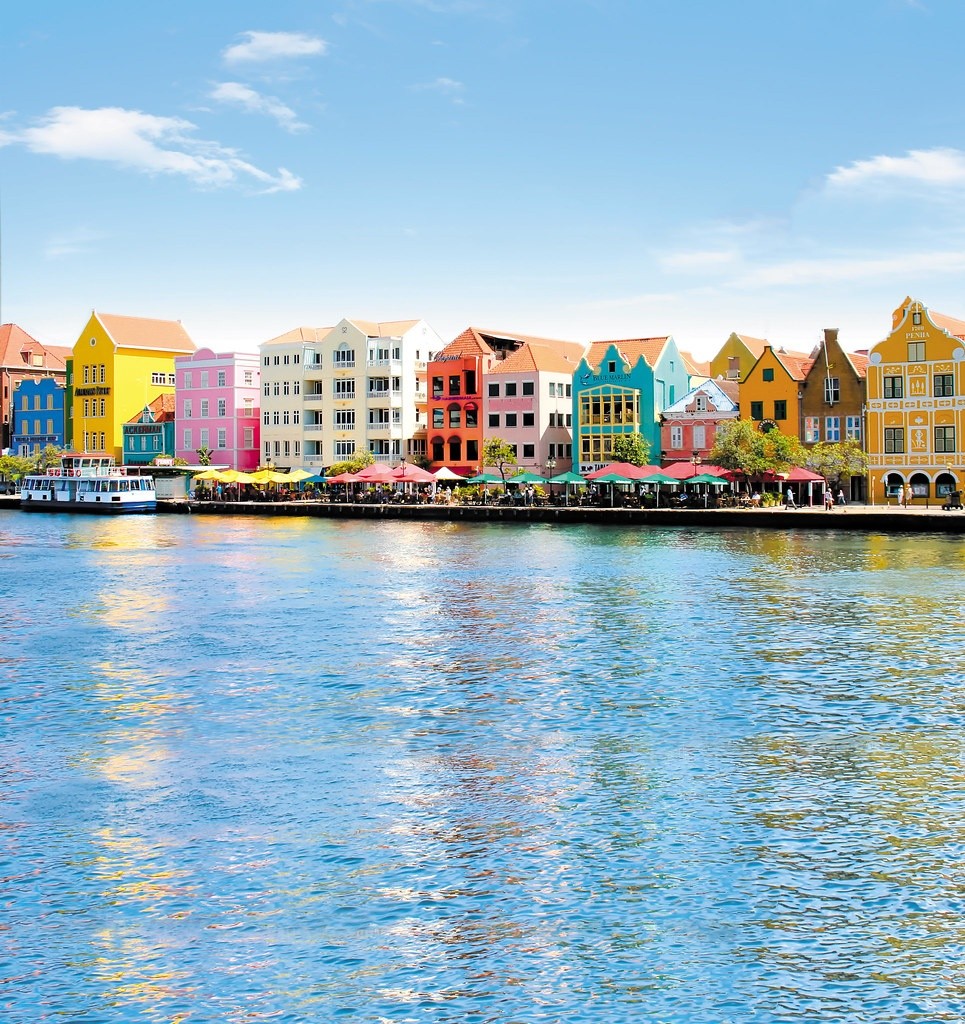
[898, 485, 904, 507]
[837, 487, 846, 505]
[195, 484, 542, 507]
[785, 486, 799, 510]
[826, 488, 834, 510]
[905, 484, 914, 505]
[548, 485, 760, 508]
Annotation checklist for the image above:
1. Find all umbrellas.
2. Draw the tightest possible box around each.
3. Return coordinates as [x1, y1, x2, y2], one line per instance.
[546, 471, 588, 506]
[506, 473, 549, 505]
[684, 473, 730, 507]
[327, 472, 363, 502]
[397, 473, 434, 501]
[466, 474, 505, 503]
[591, 473, 634, 507]
[636, 473, 681, 507]
[361, 473, 397, 495]
[194, 470, 328, 501]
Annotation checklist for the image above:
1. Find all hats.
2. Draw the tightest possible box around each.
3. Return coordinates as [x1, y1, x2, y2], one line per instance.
[900, 485, 903, 488]
[828, 488, 832, 492]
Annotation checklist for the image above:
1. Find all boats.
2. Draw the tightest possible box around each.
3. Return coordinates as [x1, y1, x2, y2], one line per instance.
[20, 455, 157, 514]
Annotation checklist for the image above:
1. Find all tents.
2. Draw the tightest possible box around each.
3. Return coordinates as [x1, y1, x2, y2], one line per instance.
[583, 461, 824, 505]
[355, 463, 468, 492]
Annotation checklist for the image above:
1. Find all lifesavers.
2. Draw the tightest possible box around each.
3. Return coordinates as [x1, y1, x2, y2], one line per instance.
[50, 470, 53, 475]
[68, 469, 74, 476]
[76, 470, 82, 477]
[55, 469, 60, 476]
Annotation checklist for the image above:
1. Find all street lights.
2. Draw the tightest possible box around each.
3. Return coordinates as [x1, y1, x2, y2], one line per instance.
[545, 456, 556, 492]
[266, 457, 271, 490]
[691, 450, 702, 492]
[401, 457, 407, 496]
